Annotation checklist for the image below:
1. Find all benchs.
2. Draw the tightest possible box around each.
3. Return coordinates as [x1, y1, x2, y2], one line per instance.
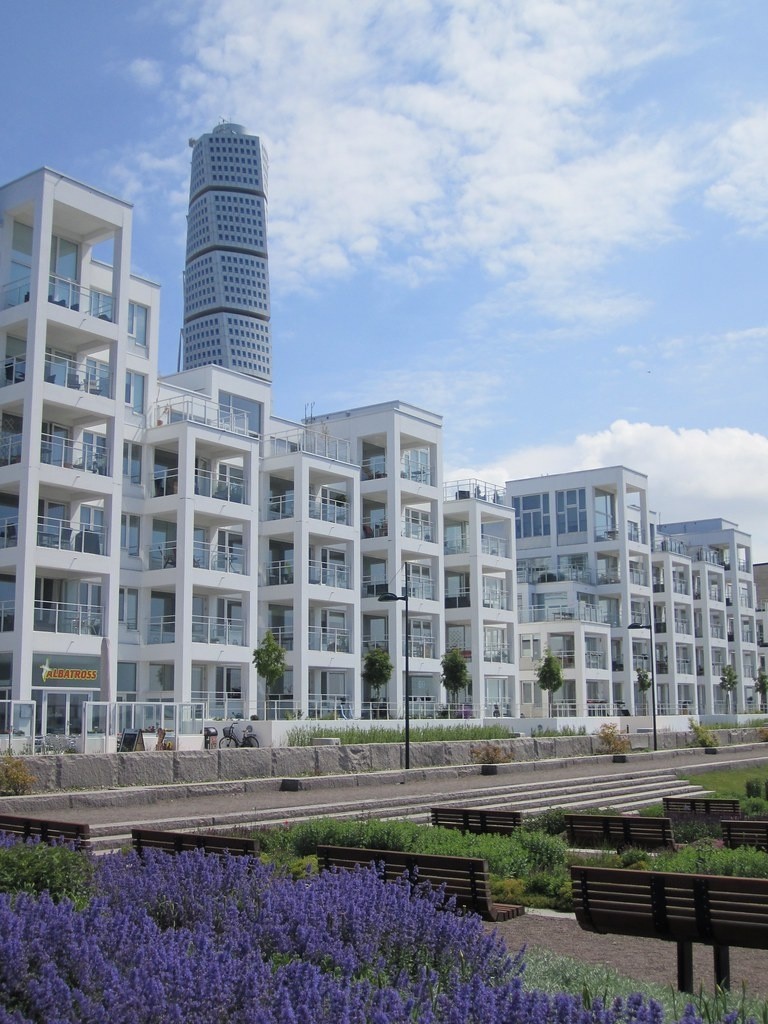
[571, 866, 768, 994]
[317, 845, 525, 922]
[132, 829, 259, 872]
[431, 807, 526, 837]
[721, 819, 768, 849]
[663, 798, 740, 817]
[0, 815, 90, 851]
[564, 814, 675, 851]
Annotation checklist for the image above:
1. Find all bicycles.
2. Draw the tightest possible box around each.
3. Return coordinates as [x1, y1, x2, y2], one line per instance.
[218, 721, 259, 748]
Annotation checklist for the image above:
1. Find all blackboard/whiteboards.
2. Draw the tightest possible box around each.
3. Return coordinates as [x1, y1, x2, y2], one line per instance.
[118, 728, 146, 752]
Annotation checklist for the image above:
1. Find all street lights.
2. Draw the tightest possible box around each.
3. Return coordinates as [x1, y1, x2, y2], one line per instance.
[627, 600, 658, 751]
[376, 561, 410, 770]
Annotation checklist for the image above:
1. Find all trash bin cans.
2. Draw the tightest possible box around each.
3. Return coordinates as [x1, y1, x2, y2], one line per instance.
[200, 727, 218, 750]
